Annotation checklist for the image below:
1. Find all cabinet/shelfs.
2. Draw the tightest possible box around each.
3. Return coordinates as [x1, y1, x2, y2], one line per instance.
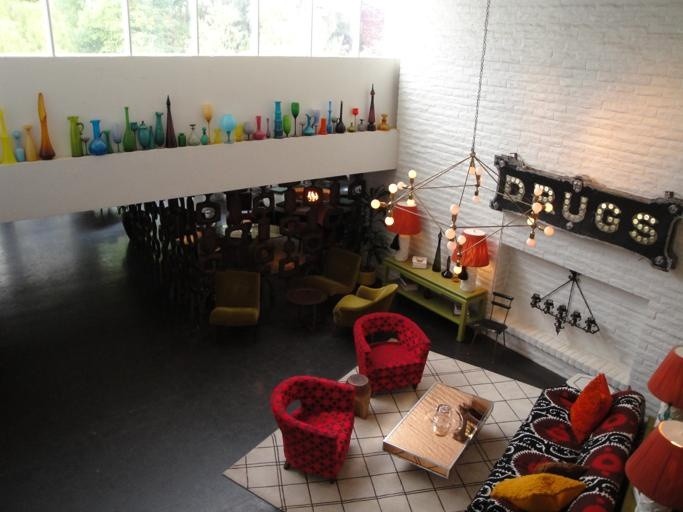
[381, 254, 492, 341]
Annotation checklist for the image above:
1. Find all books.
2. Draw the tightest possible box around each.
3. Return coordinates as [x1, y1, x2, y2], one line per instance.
[399, 276, 419, 291]
[453, 304, 471, 316]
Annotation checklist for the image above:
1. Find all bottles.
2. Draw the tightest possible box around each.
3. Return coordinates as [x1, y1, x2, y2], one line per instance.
[431, 227, 454, 280]
[433, 403, 453, 435]
[0, 84, 392, 167]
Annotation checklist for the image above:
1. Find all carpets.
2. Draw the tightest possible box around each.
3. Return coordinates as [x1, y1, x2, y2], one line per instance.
[221, 343, 546, 511]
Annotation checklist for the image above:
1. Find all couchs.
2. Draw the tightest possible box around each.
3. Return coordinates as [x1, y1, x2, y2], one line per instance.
[466, 387, 649, 512]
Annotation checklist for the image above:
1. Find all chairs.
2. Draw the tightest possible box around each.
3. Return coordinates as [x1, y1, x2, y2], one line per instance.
[157, 237, 397, 329]
[269, 374, 357, 485]
[351, 310, 431, 399]
[468, 288, 514, 348]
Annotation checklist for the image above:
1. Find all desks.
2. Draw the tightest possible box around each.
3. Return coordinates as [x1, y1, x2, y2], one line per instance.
[346, 373, 371, 419]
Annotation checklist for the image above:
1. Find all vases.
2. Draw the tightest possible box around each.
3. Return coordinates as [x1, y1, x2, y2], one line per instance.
[178, 124, 209, 146]
[300, 101, 365, 136]
[2, 92, 55, 164]
[67, 94, 177, 157]
[367, 83, 389, 131]
[254, 101, 283, 140]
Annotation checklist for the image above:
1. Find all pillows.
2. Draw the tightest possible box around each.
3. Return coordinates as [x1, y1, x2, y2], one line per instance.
[567, 373, 616, 445]
[490, 474, 590, 512]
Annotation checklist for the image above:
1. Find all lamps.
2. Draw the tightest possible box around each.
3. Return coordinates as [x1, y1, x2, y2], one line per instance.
[647, 345, 683, 415]
[622, 418, 682, 510]
[366, 2, 557, 275]
[382, 198, 423, 260]
[449, 229, 492, 294]
